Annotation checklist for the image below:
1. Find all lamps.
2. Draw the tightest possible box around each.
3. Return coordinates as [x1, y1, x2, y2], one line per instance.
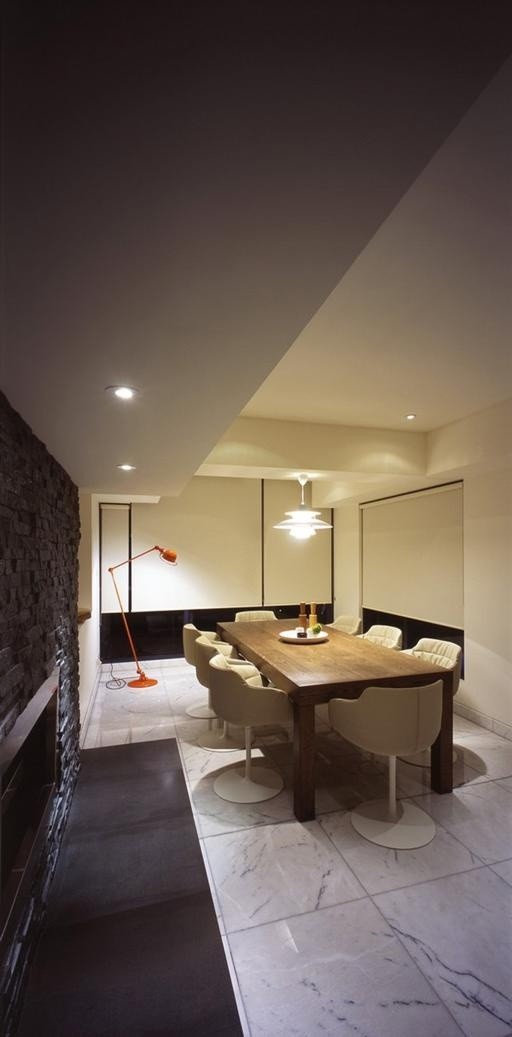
[272, 474, 334, 540]
[107, 544, 184, 688]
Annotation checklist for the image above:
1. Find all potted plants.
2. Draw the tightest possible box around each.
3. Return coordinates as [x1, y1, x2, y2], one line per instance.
[306, 622, 322, 638]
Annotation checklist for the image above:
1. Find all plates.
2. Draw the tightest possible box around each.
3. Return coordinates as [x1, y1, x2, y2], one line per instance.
[278, 629, 328, 643]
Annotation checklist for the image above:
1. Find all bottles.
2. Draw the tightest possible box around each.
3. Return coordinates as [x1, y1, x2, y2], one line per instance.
[309, 601, 318, 626]
[298, 602, 307, 628]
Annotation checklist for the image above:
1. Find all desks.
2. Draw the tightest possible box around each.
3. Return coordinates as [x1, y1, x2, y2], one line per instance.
[216, 616, 455, 823]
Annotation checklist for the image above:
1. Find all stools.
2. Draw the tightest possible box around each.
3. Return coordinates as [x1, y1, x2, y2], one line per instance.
[326, 614, 363, 636]
[397, 637, 463, 765]
[182, 620, 294, 804]
[354, 623, 404, 652]
[329, 677, 449, 859]
